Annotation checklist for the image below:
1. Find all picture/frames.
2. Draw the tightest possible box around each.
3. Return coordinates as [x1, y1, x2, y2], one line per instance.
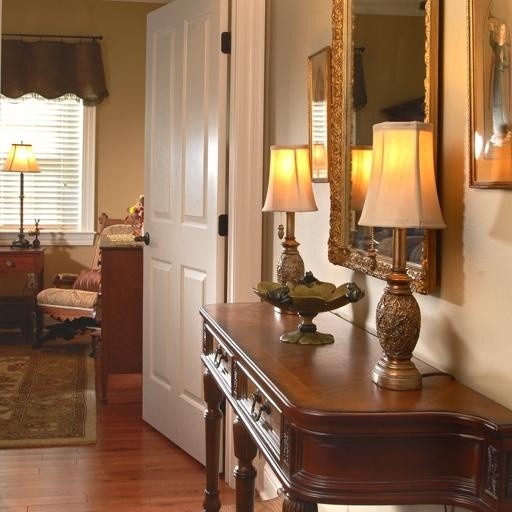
[466, 0, 512, 189]
[306, 45, 332, 183]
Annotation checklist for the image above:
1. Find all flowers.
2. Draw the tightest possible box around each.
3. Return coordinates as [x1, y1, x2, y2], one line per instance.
[125, 194, 145, 227]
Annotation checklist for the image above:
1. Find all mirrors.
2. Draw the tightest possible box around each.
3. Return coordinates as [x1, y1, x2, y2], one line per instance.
[328, 0, 441, 296]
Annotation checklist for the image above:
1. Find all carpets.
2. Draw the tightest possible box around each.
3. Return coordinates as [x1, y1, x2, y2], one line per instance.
[0, 324, 98, 450]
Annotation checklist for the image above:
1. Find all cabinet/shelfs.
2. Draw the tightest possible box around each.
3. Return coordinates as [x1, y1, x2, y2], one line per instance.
[98, 234, 143, 406]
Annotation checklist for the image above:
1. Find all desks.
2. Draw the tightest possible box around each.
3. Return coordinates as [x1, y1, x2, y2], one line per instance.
[198, 301, 512, 512]
[0, 246, 47, 347]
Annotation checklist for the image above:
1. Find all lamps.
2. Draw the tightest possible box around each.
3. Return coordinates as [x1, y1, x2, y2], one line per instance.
[348, 143, 374, 251]
[3, 141, 40, 248]
[311, 141, 327, 178]
[357, 121, 447, 393]
[261, 144, 318, 315]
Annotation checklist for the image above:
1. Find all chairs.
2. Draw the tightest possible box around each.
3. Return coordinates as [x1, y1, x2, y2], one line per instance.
[32, 212, 128, 357]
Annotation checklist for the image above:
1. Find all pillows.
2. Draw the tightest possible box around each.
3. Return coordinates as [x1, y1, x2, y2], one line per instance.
[73, 269, 102, 292]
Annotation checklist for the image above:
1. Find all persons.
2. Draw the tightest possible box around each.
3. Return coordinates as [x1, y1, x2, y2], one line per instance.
[487, 22, 511, 143]
